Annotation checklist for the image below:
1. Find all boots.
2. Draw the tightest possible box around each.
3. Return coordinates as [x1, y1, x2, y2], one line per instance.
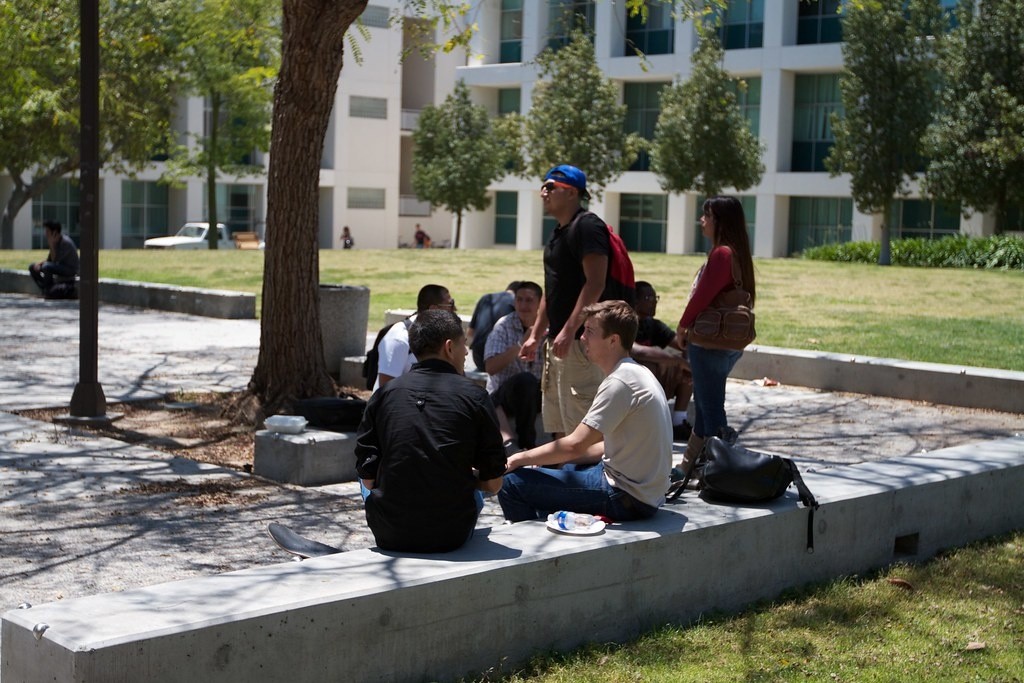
[675, 427, 703, 479]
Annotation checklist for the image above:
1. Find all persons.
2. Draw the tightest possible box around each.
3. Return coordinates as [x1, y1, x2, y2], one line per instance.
[339, 226, 354, 248]
[353, 309, 506, 555]
[627, 281, 694, 442]
[483, 283, 556, 452]
[497, 300, 674, 522]
[371, 284, 458, 395]
[413, 224, 430, 249]
[668, 196, 755, 480]
[519, 164, 631, 445]
[467, 281, 521, 373]
[29, 219, 80, 298]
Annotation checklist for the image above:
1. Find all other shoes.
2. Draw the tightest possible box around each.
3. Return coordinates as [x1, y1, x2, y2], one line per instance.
[39, 286, 47, 297]
[673, 419, 692, 440]
[517, 442, 536, 449]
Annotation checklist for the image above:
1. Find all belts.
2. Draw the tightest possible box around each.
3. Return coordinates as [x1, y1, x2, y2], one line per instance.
[613, 487, 634, 512]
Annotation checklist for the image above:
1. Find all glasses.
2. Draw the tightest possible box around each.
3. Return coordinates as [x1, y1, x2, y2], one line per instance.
[636, 296, 659, 302]
[541, 182, 578, 192]
[437, 299, 454, 308]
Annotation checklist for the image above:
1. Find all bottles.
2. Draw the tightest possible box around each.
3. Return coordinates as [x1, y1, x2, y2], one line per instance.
[547, 511, 596, 531]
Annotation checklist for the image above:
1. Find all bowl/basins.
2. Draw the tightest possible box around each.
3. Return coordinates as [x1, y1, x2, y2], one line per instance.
[263, 414, 308, 434]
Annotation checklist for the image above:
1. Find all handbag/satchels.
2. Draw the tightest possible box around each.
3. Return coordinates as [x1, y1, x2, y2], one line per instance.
[301, 393, 367, 427]
[48, 281, 77, 298]
[687, 245, 756, 349]
[700, 425, 819, 554]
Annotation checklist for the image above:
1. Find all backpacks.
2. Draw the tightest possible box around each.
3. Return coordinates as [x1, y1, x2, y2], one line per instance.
[362, 318, 411, 390]
[568, 212, 634, 305]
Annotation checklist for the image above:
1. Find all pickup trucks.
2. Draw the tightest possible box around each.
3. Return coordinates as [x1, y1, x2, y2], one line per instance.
[143, 222, 265, 251]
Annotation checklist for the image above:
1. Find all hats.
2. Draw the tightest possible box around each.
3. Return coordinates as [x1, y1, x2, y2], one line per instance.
[544, 164, 591, 200]
